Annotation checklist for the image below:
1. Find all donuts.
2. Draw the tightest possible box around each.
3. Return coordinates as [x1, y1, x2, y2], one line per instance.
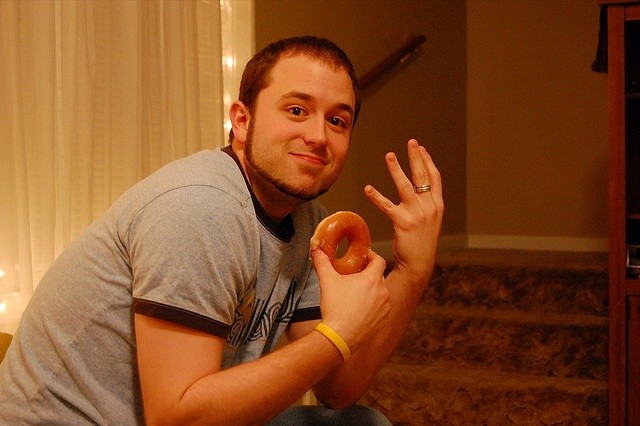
[312, 212, 372, 275]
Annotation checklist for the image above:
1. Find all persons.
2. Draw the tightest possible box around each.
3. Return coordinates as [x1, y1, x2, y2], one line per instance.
[0, 35, 445, 426]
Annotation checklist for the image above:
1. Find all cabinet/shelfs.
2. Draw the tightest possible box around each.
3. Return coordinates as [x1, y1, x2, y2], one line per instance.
[599, 1, 640, 426]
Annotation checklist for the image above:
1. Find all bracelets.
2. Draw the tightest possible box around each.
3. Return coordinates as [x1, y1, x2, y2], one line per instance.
[315, 322, 352, 362]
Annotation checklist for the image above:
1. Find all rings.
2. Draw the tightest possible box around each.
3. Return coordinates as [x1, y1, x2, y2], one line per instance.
[414, 185, 432, 193]
